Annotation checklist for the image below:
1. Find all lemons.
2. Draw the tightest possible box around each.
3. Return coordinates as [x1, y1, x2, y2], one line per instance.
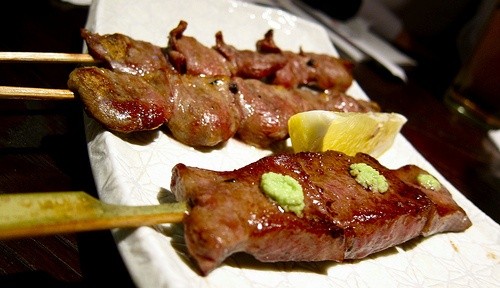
[288, 110, 408, 159]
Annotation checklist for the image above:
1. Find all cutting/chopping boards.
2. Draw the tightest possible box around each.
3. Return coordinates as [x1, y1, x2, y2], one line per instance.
[82, 0, 500, 288]
